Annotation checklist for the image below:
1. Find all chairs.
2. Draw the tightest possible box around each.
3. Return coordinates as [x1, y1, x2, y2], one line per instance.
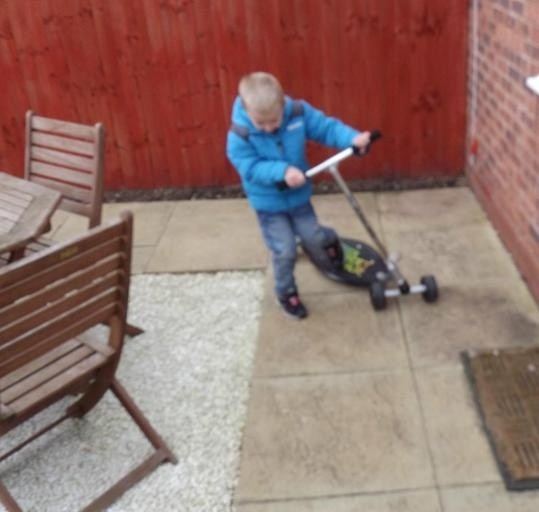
[2, 109, 105, 263]
[1, 210, 179, 511]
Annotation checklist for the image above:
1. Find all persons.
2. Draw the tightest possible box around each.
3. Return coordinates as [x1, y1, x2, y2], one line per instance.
[225, 72, 371, 319]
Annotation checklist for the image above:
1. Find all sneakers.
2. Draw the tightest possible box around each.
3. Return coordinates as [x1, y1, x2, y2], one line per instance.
[280, 291, 307, 320]
[326, 227, 344, 273]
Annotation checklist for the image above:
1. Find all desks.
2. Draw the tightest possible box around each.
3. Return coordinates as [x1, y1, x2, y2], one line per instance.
[0, 174, 62, 263]
